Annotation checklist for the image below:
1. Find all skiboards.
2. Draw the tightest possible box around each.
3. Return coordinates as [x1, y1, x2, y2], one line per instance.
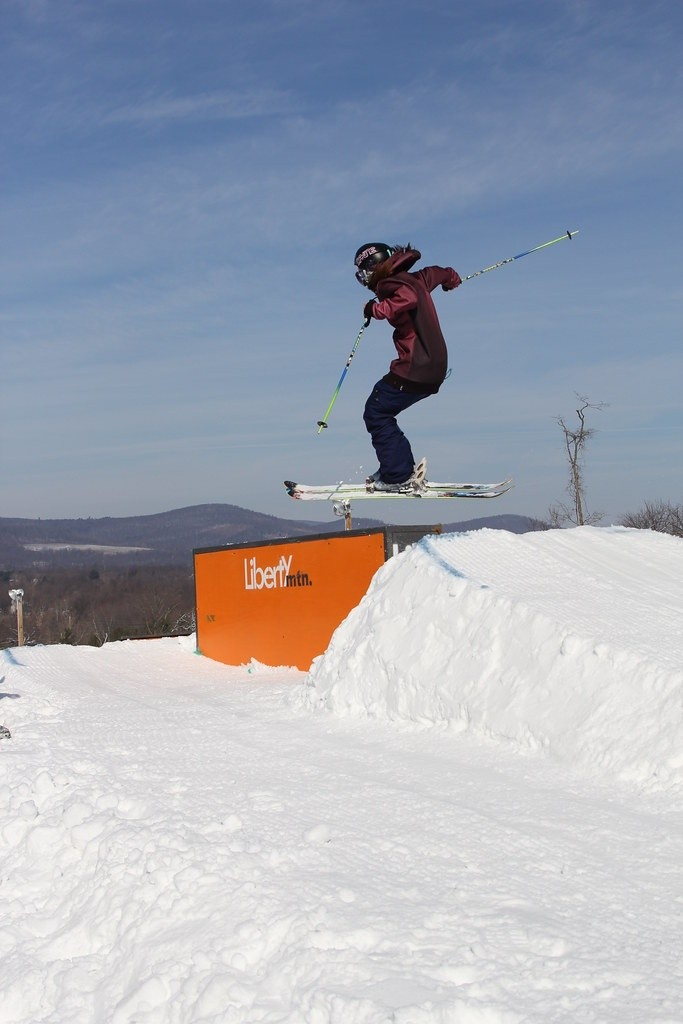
[284, 480, 515, 501]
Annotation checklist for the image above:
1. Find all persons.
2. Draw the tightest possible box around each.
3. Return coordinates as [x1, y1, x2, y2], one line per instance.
[354, 242, 462, 491]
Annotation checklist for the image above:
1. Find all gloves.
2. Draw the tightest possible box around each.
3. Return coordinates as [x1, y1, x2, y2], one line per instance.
[364, 300, 376, 318]
[443, 280, 462, 291]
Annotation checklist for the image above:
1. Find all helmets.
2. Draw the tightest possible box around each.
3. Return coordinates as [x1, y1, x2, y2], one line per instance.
[354, 242, 392, 276]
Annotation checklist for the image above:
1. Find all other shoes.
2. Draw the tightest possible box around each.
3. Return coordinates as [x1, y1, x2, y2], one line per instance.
[369, 465, 416, 491]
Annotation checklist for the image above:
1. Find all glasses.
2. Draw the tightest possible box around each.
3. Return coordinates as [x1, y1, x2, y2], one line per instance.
[355, 271, 367, 286]
